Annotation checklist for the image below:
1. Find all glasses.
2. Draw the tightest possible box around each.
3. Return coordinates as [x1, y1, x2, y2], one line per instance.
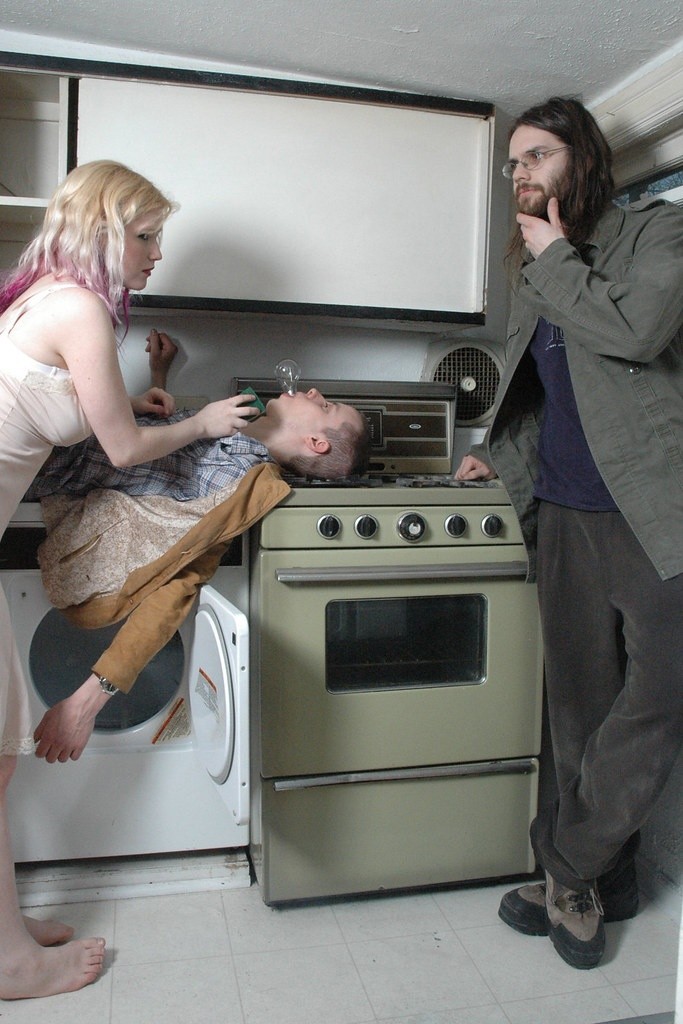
[502, 144, 572, 181]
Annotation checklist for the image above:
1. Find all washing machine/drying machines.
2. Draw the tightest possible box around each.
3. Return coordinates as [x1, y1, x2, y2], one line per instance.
[0, 500, 264, 862]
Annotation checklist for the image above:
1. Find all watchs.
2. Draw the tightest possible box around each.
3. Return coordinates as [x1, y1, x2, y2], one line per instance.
[93, 671, 119, 696]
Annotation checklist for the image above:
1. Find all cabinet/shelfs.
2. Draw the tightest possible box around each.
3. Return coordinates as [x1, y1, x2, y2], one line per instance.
[0, 50, 499, 332]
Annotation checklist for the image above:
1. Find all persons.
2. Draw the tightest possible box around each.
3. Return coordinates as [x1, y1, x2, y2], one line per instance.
[19, 324, 373, 766]
[448, 94, 682, 971]
[0, 158, 262, 1000]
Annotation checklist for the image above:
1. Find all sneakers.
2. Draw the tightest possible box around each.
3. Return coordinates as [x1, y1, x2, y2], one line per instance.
[496, 870, 639, 972]
